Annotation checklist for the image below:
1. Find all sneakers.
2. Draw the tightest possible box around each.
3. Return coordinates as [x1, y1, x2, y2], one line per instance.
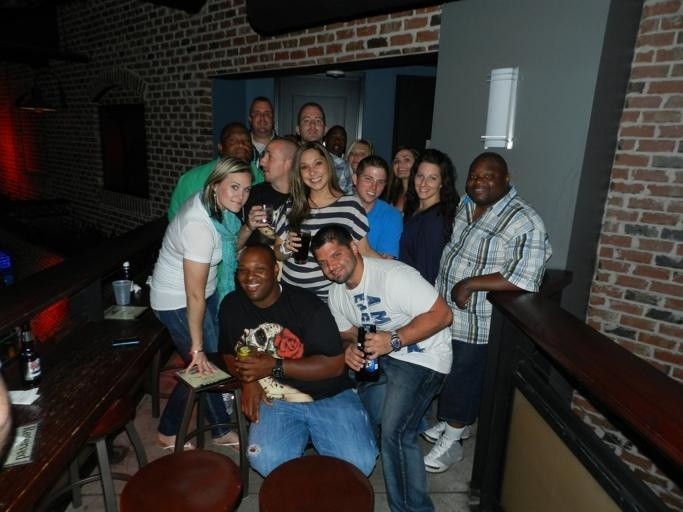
[419, 434, 466, 473]
[221, 392, 235, 416]
[419, 419, 473, 443]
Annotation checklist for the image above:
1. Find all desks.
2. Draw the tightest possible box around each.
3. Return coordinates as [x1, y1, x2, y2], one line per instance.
[0, 300, 170, 512]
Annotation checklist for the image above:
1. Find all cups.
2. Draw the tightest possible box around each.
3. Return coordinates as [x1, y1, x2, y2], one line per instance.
[112, 279, 132, 306]
[293, 228, 311, 266]
[262, 204, 274, 226]
[233, 341, 260, 358]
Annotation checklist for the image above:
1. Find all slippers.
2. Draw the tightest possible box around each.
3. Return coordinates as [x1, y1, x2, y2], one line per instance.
[211, 434, 241, 446]
[154, 436, 195, 455]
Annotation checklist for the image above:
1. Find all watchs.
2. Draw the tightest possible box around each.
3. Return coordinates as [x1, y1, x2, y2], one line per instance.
[390, 329, 402, 353]
[272, 358, 284, 377]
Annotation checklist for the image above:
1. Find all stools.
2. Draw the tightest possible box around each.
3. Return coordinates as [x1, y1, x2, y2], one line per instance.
[175, 353, 249, 499]
[68, 389, 150, 512]
[258, 453, 374, 512]
[150, 326, 194, 418]
[119, 449, 245, 512]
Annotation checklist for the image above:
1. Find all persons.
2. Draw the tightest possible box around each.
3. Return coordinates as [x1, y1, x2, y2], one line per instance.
[344, 138, 372, 171]
[168, 122, 265, 305]
[295, 101, 356, 196]
[322, 126, 350, 156]
[382, 142, 419, 208]
[421, 152, 552, 473]
[221, 245, 380, 480]
[400, 150, 460, 285]
[311, 226, 454, 507]
[267, 141, 394, 305]
[150, 157, 254, 453]
[346, 157, 403, 257]
[246, 98, 278, 162]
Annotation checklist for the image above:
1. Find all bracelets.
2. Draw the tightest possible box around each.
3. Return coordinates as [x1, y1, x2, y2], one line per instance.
[278, 241, 291, 255]
[189, 348, 203, 354]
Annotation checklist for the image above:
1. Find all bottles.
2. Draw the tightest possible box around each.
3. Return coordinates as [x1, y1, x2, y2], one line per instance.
[18, 315, 41, 388]
[123, 261, 134, 298]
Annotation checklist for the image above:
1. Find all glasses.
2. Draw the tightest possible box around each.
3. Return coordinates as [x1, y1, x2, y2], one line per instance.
[357, 174, 387, 185]
